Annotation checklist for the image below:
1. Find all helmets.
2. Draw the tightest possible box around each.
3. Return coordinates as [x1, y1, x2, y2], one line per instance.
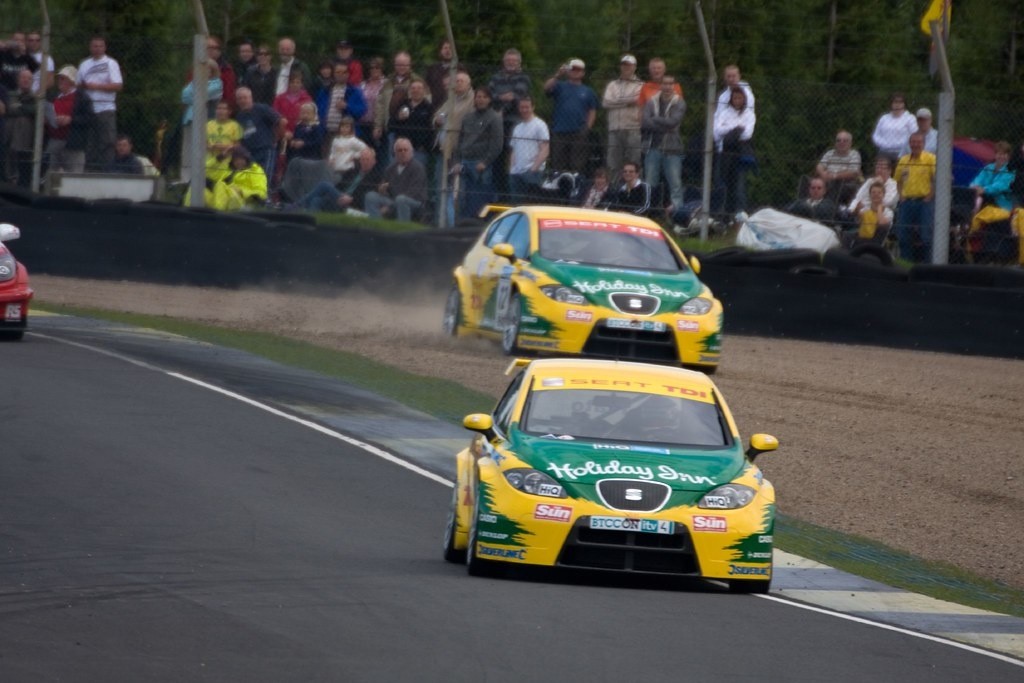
[632, 393, 682, 432]
[916, 108, 932, 119]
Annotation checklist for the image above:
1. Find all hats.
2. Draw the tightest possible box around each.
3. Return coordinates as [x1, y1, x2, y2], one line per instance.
[57, 65, 78, 86]
[620, 54, 637, 64]
[568, 59, 586, 70]
[335, 39, 352, 48]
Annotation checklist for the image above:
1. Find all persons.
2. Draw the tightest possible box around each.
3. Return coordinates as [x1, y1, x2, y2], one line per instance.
[640, 396, 683, 432]
[504, 95, 549, 205]
[712, 64, 756, 220]
[76, 34, 122, 172]
[0, 29, 94, 183]
[582, 55, 686, 215]
[544, 59, 598, 172]
[102, 134, 144, 174]
[490, 48, 531, 180]
[787, 93, 1024, 264]
[156, 35, 502, 225]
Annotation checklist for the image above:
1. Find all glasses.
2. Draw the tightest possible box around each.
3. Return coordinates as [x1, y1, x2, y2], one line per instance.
[398, 148, 409, 152]
[624, 170, 634, 173]
[28, 39, 40, 42]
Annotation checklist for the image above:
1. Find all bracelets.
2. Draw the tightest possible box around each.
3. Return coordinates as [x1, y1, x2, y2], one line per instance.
[554, 73, 561, 80]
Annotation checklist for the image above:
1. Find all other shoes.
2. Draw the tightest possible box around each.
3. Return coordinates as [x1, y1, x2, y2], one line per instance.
[735, 210, 748, 223]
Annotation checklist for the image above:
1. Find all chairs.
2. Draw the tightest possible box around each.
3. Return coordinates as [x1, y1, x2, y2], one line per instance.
[795, 173, 845, 226]
[952, 185, 977, 247]
[282, 157, 336, 202]
[672, 185, 728, 228]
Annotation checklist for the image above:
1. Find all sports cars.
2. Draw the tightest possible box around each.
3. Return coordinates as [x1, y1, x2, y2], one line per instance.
[440, 358, 783, 595]
[442, 204, 725, 374]
[0, 222, 33, 338]
